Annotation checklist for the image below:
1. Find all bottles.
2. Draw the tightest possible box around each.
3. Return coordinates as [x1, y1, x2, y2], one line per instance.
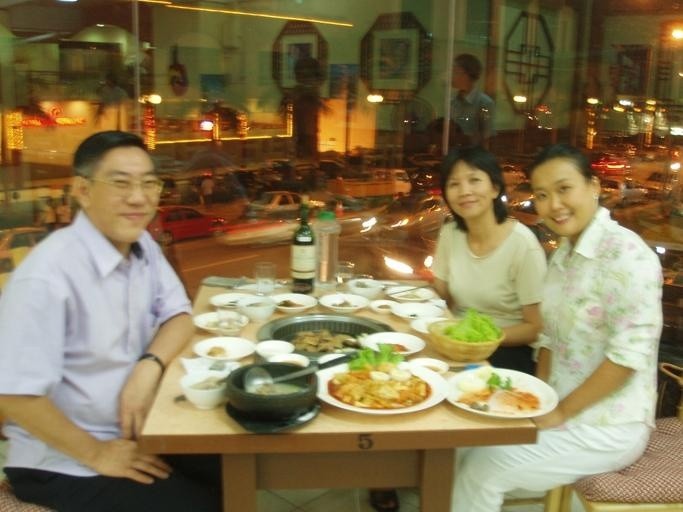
[312, 210, 340, 284]
[288, 201, 317, 294]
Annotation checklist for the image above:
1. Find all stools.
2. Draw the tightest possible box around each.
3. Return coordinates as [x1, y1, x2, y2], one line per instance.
[544, 412, 683, 512]
[0, 479, 58, 512]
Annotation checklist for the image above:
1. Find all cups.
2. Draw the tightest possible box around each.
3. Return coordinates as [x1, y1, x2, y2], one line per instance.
[252, 260, 276, 297]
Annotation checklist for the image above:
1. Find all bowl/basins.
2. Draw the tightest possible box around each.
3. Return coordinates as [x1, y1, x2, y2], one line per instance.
[179, 370, 224, 411]
[224, 361, 317, 416]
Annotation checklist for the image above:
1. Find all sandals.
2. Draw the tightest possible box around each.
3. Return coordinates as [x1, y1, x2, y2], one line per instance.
[370, 488, 399, 512]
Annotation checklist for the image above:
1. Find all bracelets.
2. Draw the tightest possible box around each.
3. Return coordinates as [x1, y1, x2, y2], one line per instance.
[135, 353, 165, 372]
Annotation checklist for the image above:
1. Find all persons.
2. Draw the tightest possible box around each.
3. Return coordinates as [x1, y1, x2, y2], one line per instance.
[100, 76, 128, 110]
[451, 143, 665, 511]
[24, 160, 429, 234]
[427, 151, 550, 380]
[439, 52, 495, 161]
[0, 132, 222, 509]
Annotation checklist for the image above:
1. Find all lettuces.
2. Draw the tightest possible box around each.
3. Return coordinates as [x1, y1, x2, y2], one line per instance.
[442, 309, 501, 343]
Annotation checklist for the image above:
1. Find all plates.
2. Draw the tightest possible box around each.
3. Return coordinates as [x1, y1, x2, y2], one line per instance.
[320, 277, 448, 323]
[314, 361, 448, 415]
[256, 338, 310, 367]
[441, 367, 560, 418]
[191, 336, 256, 361]
[362, 330, 425, 356]
[191, 290, 316, 334]
[411, 355, 448, 374]
[410, 315, 443, 336]
[184, 356, 241, 378]
[234, 282, 282, 296]
[225, 395, 320, 434]
[316, 353, 346, 366]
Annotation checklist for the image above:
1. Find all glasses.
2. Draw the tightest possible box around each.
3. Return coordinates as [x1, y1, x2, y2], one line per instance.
[91, 176, 165, 195]
